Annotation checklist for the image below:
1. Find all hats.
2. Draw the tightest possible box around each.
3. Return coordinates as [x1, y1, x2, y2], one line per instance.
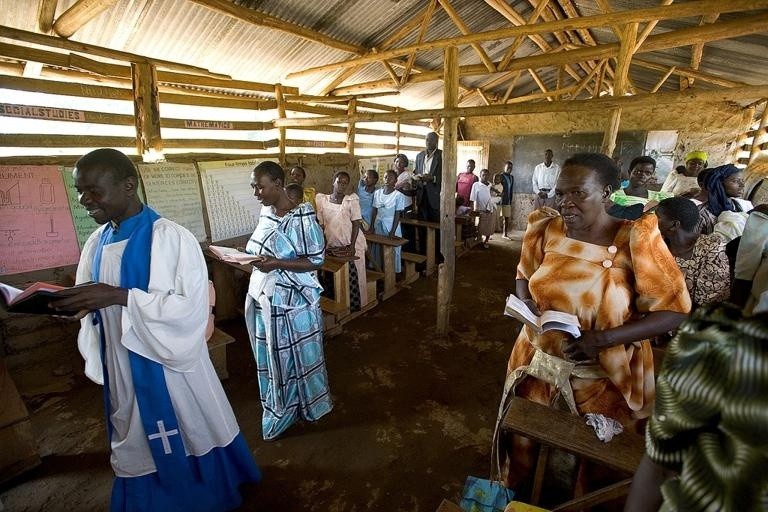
[684, 151, 707, 165]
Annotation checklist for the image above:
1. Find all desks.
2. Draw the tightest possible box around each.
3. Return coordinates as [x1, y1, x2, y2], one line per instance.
[501, 397, 644, 511]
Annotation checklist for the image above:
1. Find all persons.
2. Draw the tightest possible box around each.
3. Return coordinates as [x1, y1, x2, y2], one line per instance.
[456, 159, 518, 248]
[286, 155, 411, 310]
[413, 131, 447, 268]
[532, 150, 561, 208]
[625, 289, 767, 512]
[48, 145, 258, 508]
[612, 145, 768, 317]
[487, 151, 692, 512]
[236, 160, 337, 441]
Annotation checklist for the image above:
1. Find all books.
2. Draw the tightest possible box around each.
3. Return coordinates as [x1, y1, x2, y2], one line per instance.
[411, 171, 425, 181]
[504, 291, 582, 340]
[0, 280, 87, 323]
[207, 242, 264, 266]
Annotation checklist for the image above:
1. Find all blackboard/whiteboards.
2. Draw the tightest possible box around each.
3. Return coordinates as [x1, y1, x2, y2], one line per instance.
[511, 129, 648, 193]
